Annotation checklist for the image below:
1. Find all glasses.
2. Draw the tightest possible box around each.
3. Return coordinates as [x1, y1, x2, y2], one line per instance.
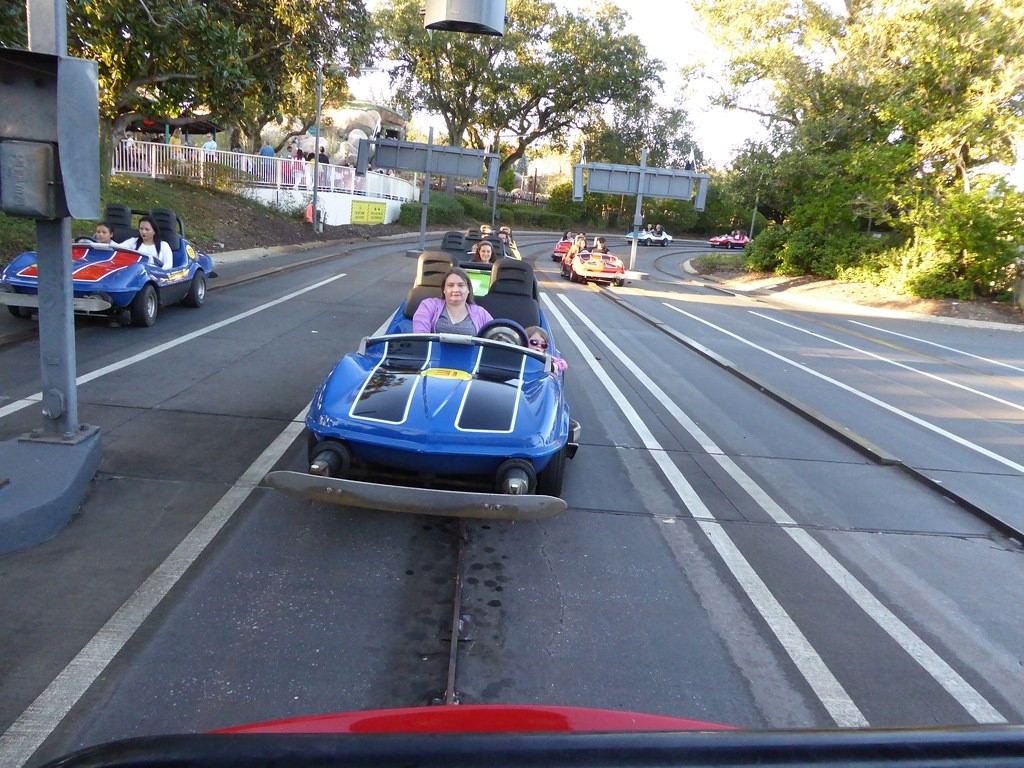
[481, 232, 492, 236]
[530, 339, 548, 350]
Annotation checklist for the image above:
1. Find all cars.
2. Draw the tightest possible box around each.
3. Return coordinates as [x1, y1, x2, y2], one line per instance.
[708, 234, 755, 249]
[626, 229, 674, 246]
[0, 203, 218, 329]
[264, 227, 581, 522]
[549, 240, 629, 288]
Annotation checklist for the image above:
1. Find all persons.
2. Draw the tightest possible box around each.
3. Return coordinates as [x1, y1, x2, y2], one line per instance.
[96, 222, 118, 244]
[114, 216, 173, 271]
[728, 230, 744, 242]
[560, 229, 611, 262]
[642, 223, 662, 237]
[412, 266, 495, 338]
[466, 227, 522, 265]
[524, 326, 568, 375]
[166, 127, 396, 201]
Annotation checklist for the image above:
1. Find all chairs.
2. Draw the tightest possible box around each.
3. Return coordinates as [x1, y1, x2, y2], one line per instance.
[441, 229, 509, 260]
[104, 203, 132, 243]
[150, 207, 180, 249]
[482, 258, 540, 327]
[403, 251, 453, 318]
